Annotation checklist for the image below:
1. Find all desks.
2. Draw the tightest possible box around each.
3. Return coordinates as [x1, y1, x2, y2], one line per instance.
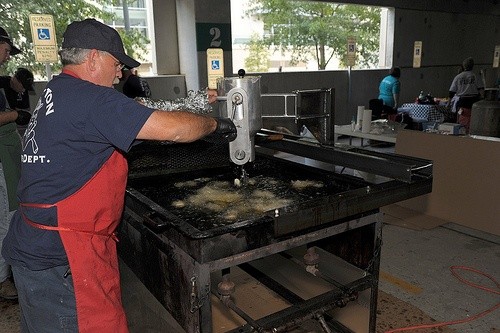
[400, 103, 446, 131]
[333, 124, 397, 146]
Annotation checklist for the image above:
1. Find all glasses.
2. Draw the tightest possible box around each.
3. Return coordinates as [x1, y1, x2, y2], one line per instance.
[99, 50, 125, 71]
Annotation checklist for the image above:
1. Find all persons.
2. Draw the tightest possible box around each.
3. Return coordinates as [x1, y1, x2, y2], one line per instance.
[1, 67, 35, 109]
[446, 57, 484, 131]
[378, 67, 401, 122]
[121, 66, 153, 99]
[0, 26, 32, 299]
[0, 19, 239, 332]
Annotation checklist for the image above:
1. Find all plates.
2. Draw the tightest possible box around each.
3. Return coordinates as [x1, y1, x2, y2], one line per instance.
[369, 122, 407, 132]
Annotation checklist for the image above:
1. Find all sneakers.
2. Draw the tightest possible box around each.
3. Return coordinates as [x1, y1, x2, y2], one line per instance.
[0, 279, 18, 300]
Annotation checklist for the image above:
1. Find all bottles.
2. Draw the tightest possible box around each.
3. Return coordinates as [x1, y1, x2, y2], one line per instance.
[421, 91, 424, 98]
[418, 94, 423, 99]
[351, 114, 356, 131]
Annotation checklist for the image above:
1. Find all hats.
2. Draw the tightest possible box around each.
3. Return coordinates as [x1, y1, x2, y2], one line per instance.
[0, 27, 21, 55]
[14, 68, 35, 91]
[61, 18, 140, 69]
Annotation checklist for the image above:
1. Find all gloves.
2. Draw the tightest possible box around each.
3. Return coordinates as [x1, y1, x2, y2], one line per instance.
[14, 110, 32, 126]
[202, 117, 237, 145]
